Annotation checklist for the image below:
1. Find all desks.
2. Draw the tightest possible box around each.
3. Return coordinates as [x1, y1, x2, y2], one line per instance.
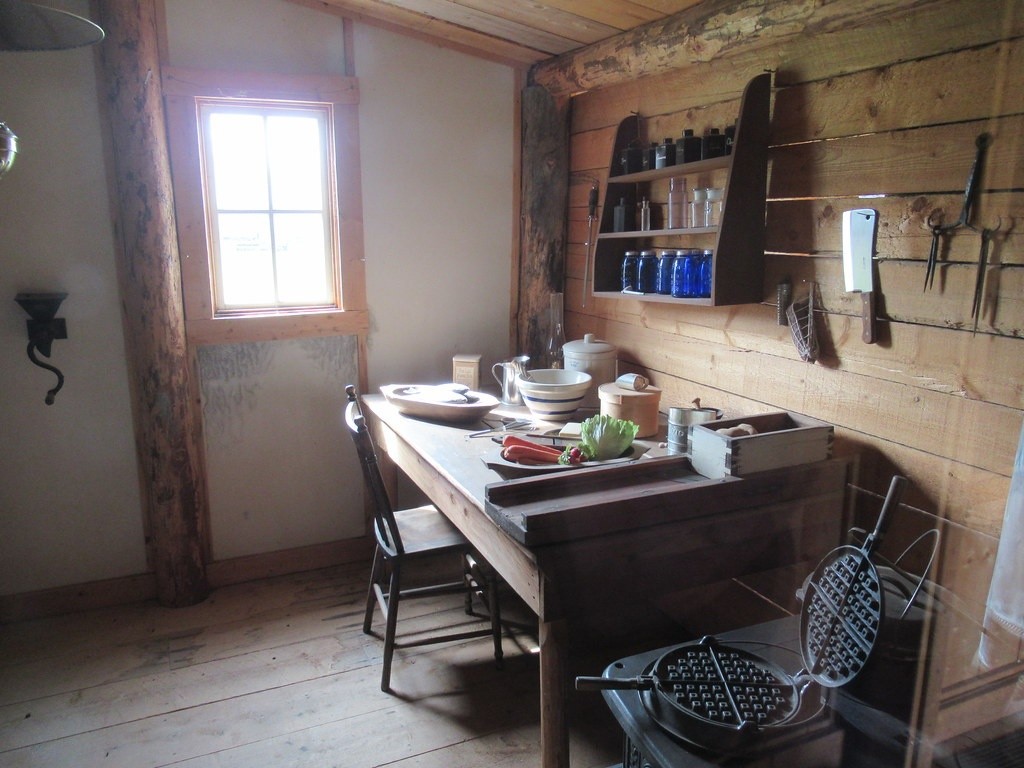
[359, 393, 854, 768]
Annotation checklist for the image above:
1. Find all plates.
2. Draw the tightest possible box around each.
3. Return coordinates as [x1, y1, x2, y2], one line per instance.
[378, 384, 502, 421]
[480, 440, 652, 481]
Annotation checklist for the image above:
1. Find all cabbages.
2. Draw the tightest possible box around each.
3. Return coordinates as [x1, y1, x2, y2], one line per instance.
[579, 414, 640, 460]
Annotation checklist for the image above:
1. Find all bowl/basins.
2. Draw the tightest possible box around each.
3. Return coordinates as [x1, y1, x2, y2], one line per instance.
[514, 369, 592, 421]
[693, 407, 723, 420]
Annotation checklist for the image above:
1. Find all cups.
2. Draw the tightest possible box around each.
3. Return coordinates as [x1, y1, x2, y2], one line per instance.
[546, 320, 564, 369]
[561, 333, 617, 408]
[491, 355, 530, 406]
[615, 372, 649, 392]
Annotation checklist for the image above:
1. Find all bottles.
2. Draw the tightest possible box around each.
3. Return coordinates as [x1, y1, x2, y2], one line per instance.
[617, 248, 713, 298]
[641, 200, 651, 231]
[613, 197, 635, 232]
[617, 127, 727, 175]
[667, 177, 688, 229]
[690, 187, 725, 227]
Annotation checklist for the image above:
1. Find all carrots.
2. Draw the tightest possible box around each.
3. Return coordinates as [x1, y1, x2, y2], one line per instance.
[503, 435, 563, 462]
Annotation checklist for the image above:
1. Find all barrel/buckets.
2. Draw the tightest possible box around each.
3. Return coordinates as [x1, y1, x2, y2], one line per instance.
[597, 382, 662, 438]
[667, 407, 716, 455]
[832, 528, 966, 717]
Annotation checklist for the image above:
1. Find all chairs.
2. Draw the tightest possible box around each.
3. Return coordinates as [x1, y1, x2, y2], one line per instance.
[345, 384, 504, 690]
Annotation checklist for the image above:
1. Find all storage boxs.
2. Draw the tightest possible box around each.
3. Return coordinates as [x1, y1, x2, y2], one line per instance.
[692, 413, 833, 480]
[452, 352, 481, 391]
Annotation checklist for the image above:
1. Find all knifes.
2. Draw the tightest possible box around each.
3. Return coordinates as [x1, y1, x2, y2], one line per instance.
[841, 208, 880, 346]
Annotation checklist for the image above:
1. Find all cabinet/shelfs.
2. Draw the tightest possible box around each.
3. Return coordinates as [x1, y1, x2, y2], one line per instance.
[590, 73, 771, 307]
